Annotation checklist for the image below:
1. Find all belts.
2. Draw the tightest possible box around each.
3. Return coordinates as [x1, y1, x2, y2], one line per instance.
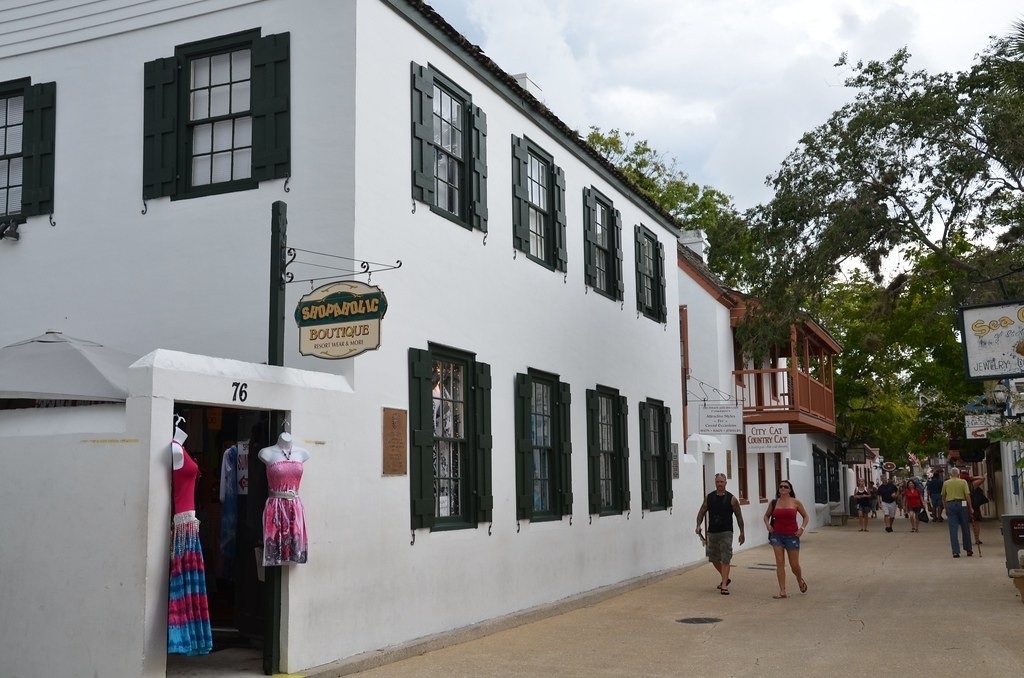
[949, 499, 965, 502]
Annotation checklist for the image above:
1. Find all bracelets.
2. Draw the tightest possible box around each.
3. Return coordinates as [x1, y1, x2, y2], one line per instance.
[801, 527, 804, 531]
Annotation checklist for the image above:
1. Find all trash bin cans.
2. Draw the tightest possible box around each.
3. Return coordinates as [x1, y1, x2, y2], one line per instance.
[849, 495, 859, 517]
[1000, 514, 1024, 579]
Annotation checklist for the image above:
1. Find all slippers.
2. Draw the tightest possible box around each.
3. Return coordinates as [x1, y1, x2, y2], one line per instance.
[717, 578, 731, 589]
[721, 589, 730, 595]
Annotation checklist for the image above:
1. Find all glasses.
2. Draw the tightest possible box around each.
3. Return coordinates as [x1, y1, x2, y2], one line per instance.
[778, 485, 789, 489]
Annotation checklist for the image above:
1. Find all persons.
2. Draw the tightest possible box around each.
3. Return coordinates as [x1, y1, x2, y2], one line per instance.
[966, 476, 989, 544]
[695, 473, 745, 595]
[903, 480, 925, 532]
[877, 475, 898, 532]
[854, 479, 872, 532]
[764, 480, 809, 598]
[941, 467, 974, 558]
[167, 427, 213, 656]
[868, 471, 945, 522]
[258, 430, 310, 566]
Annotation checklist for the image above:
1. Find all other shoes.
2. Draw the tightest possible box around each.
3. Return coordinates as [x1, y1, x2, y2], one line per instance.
[886, 527, 893, 532]
[772, 591, 787, 599]
[911, 527, 919, 532]
[798, 577, 809, 593]
[858, 529, 869, 532]
[953, 553, 959, 558]
[967, 551, 973, 556]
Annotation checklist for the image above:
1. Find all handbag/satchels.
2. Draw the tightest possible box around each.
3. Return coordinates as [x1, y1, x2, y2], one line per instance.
[970, 481, 989, 508]
[768, 499, 777, 540]
[918, 508, 929, 523]
